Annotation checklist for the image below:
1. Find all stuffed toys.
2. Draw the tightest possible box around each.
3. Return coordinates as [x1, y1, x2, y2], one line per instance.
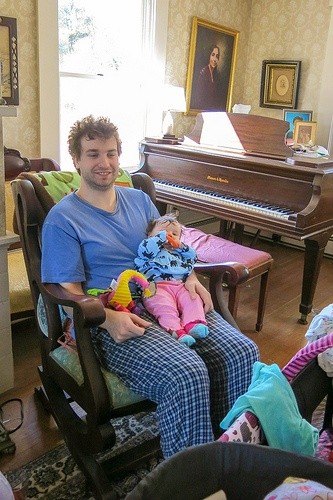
[96, 269, 149, 316]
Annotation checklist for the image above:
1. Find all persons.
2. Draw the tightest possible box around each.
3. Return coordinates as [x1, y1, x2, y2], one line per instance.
[134, 216, 210, 346]
[287, 116, 304, 139]
[41, 115, 261, 461]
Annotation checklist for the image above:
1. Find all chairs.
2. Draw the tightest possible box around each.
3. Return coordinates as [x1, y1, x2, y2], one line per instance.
[10, 172, 242, 500]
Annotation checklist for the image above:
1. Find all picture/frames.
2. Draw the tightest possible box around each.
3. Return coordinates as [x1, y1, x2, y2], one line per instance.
[0, 16, 20, 106]
[183, 16, 240, 116]
[294, 120, 317, 150]
[259, 59, 301, 110]
[284, 110, 313, 146]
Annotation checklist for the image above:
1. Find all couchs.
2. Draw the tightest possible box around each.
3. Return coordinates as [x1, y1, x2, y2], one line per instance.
[4, 146, 61, 323]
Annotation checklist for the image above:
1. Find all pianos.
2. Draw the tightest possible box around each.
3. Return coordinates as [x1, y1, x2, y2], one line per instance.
[130, 112, 333, 325]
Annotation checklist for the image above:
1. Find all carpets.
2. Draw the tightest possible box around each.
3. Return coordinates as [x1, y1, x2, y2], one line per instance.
[5, 413, 164, 500]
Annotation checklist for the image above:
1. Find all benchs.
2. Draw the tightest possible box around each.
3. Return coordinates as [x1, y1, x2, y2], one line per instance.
[180, 224, 273, 332]
